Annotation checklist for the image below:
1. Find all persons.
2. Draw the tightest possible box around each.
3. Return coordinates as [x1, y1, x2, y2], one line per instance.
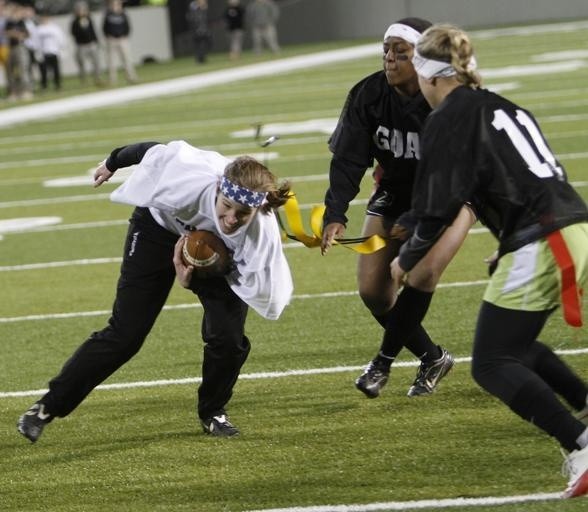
[217, 0, 247, 61]
[388, 22, 588, 497]
[246, 1, 282, 60]
[0, 0, 140, 105]
[15, 140, 297, 444]
[189, 0, 216, 65]
[321, 17, 466, 399]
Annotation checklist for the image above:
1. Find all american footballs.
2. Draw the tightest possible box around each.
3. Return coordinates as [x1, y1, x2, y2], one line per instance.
[181, 231, 226, 281]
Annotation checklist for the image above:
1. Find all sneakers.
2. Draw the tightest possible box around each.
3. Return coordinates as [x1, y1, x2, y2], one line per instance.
[199, 414, 240, 437]
[356, 361, 388, 399]
[407, 345, 455, 397]
[16, 401, 51, 443]
[561, 446, 588, 499]
